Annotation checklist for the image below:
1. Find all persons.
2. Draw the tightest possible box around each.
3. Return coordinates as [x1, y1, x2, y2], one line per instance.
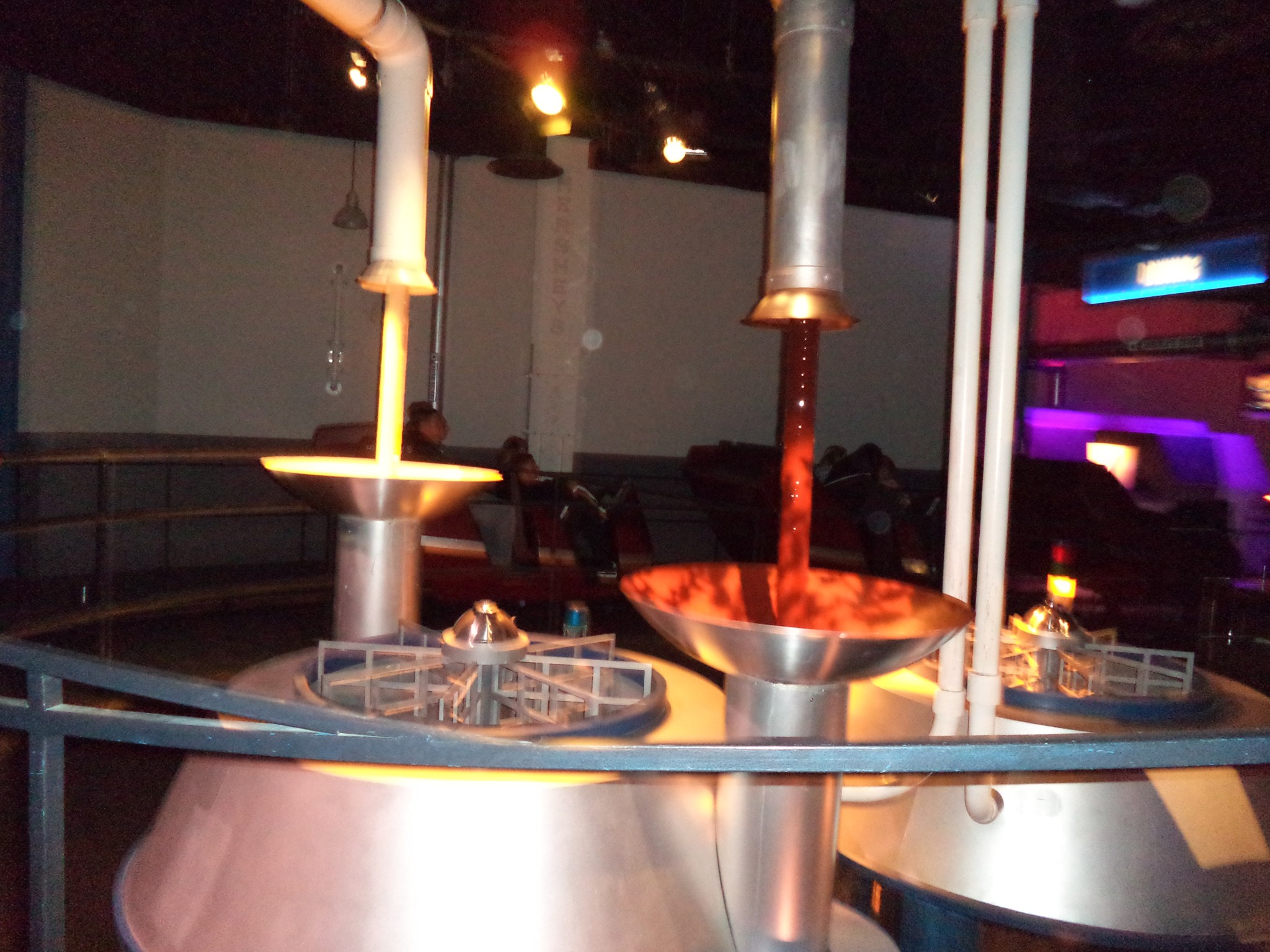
[507, 453, 617, 580]
[401, 401, 433, 458]
[814, 442, 948, 587]
[402, 409, 450, 463]
[493, 436, 529, 507]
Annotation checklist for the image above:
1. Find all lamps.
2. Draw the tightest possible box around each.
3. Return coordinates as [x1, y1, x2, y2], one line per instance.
[331, 92, 370, 231]
[488, 114, 565, 180]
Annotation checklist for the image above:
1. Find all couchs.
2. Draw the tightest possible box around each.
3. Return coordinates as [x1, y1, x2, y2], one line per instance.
[1007, 456, 1196, 626]
[421, 476, 653, 613]
[686, 439, 943, 583]
